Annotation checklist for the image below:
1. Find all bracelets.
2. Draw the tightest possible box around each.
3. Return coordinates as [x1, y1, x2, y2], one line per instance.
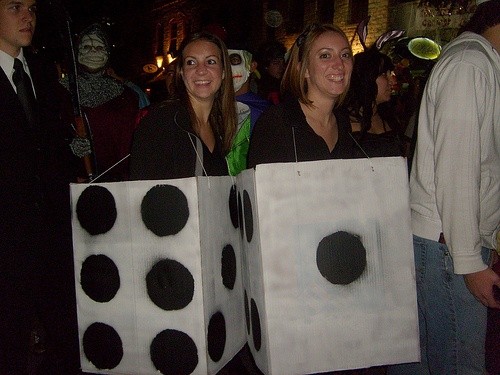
[404, 136, 414, 144]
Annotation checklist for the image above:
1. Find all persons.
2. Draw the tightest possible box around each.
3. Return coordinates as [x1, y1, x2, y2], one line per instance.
[336, 50, 415, 159]
[0, 0, 88, 375]
[59, 26, 150, 184]
[245, 22, 360, 170]
[166, 38, 288, 132]
[386, 0, 500, 375]
[125, 31, 250, 181]
